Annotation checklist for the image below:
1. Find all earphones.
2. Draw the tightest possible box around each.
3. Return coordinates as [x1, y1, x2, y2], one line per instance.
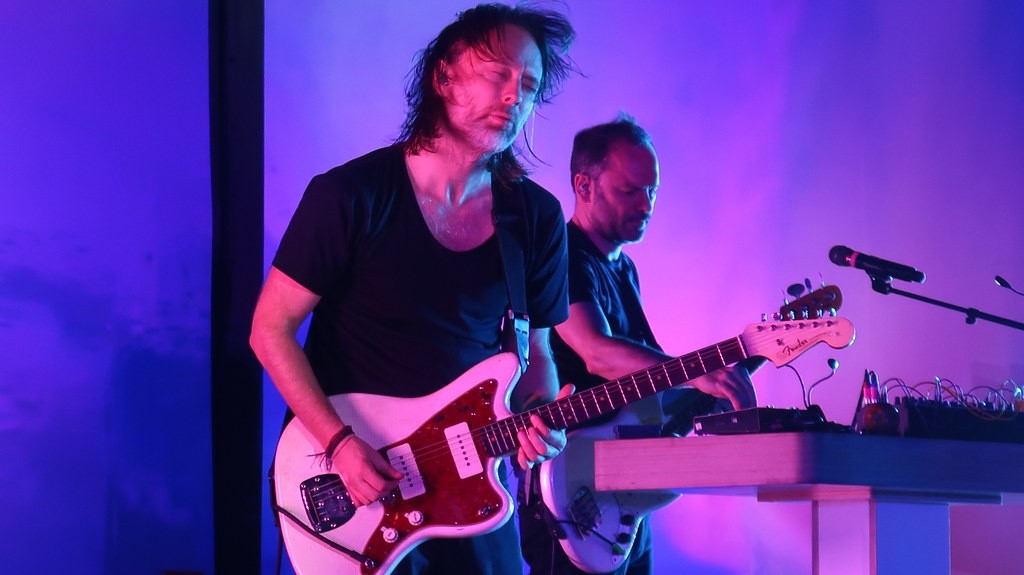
[580, 182, 588, 196]
[438, 72, 449, 86]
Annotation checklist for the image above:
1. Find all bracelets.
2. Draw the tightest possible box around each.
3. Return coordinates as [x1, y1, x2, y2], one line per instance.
[308, 425, 355, 470]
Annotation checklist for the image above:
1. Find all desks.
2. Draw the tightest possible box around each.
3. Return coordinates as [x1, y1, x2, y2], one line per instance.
[596, 431, 1024, 575]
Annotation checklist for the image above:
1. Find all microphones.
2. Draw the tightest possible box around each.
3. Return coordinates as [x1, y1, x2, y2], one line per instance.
[869, 371, 880, 404]
[829, 246, 926, 285]
[863, 369, 869, 408]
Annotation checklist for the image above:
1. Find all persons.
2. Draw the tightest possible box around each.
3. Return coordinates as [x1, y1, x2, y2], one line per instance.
[249, 3, 576, 575]
[548, 111, 756, 575]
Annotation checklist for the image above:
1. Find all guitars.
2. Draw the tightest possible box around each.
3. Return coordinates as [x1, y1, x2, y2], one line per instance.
[269, 308, 859, 575]
[520, 274, 845, 574]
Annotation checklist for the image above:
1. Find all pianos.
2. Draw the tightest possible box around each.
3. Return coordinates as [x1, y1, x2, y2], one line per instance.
[592, 400, 1024, 495]
